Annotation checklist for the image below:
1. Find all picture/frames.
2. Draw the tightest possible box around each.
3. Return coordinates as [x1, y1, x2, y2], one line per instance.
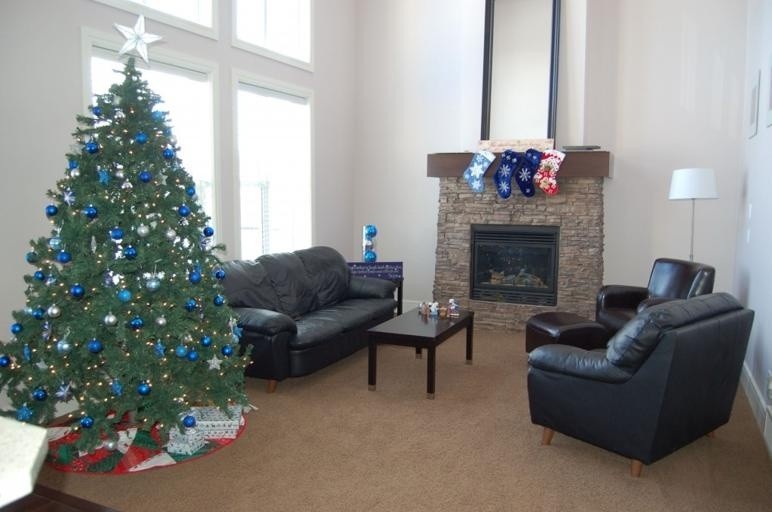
[746, 70, 760, 137]
[766, 65, 772, 128]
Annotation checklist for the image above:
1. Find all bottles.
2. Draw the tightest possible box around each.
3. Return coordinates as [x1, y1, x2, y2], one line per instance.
[447, 299, 455, 318]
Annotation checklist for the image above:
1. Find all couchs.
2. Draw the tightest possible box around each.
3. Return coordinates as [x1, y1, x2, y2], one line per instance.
[211, 246, 399, 393]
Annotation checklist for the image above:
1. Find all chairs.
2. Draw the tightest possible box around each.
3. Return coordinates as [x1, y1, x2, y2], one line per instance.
[596, 259, 715, 337]
[527, 293, 754, 477]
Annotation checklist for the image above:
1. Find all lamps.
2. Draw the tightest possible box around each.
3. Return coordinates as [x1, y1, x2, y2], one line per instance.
[668, 168, 719, 261]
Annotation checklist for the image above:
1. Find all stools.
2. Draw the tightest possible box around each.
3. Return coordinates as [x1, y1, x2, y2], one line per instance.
[526, 312, 605, 354]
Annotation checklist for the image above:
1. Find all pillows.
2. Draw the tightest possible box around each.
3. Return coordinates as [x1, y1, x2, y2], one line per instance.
[606, 292, 744, 367]
[211, 246, 350, 314]
